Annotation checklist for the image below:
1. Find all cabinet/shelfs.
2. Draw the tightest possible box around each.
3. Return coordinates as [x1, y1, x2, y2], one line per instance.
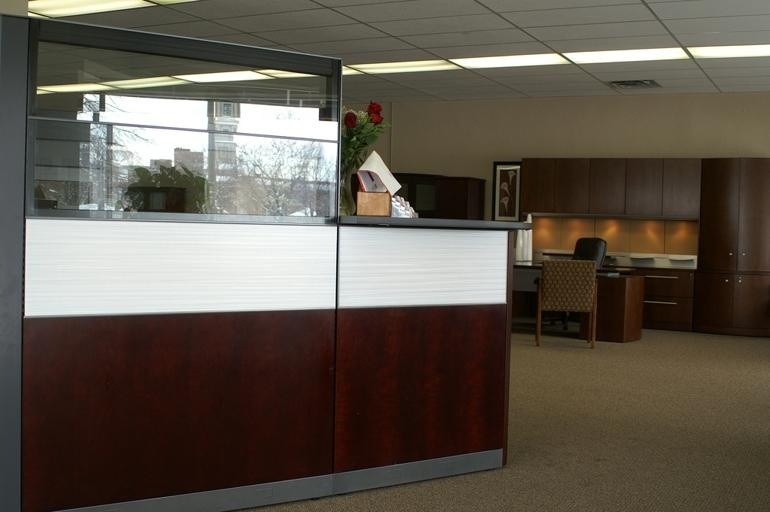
[438, 177, 485, 219]
[704, 157, 769, 274]
[633, 269, 696, 335]
[698, 270, 768, 334]
[590, 158, 700, 218]
[519, 157, 589, 214]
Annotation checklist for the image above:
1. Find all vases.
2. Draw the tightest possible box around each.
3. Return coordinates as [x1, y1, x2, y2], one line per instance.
[340, 163, 356, 216]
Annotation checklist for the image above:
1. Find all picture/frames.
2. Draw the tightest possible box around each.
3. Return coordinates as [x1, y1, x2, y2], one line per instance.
[491, 161, 521, 221]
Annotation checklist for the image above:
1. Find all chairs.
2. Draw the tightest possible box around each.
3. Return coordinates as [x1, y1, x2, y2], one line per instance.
[535, 259, 597, 348]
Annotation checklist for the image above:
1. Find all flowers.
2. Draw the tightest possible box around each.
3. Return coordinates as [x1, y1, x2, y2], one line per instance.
[340, 98, 394, 215]
